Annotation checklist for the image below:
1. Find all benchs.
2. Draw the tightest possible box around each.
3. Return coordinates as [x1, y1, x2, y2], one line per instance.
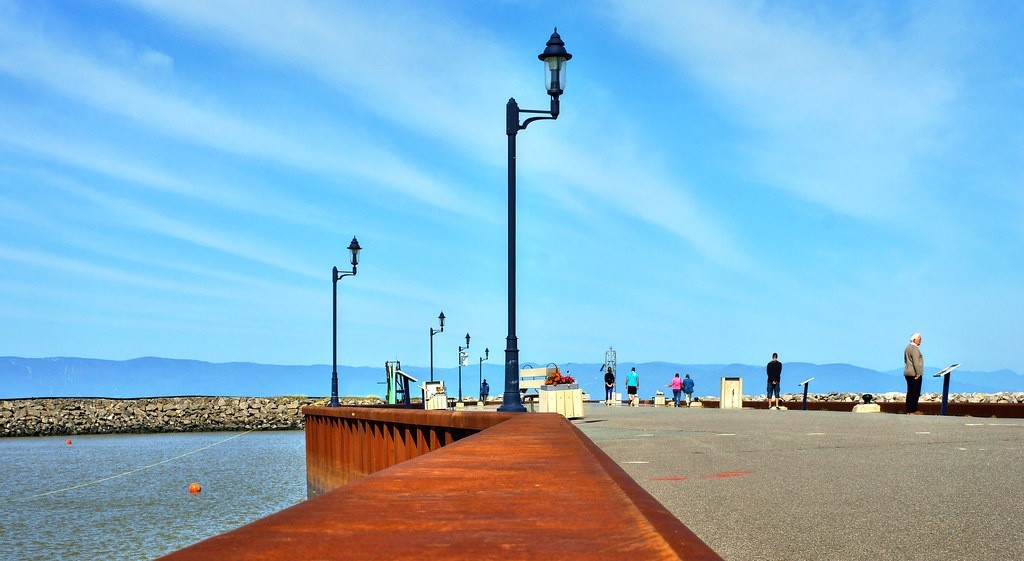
[518, 364, 560, 413]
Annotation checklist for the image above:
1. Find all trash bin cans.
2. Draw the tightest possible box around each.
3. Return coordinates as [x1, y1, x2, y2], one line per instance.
[720, 377, 743, 409]
[423, 380, 444, 399]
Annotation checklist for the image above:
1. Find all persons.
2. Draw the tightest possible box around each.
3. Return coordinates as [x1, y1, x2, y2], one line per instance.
[603, 367, 615, 405]
[625, 366, 639, 406]
[667, 373, 684, 407]
[765, 353, 782, 410]
[480, 378, 490, 403]
[682, 373, 695, 407]
[903, 332, 925, 416]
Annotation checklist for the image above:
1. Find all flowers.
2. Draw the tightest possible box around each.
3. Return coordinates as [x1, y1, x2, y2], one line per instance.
[546, 371, 575, 385]
[435, 386, 447, 393]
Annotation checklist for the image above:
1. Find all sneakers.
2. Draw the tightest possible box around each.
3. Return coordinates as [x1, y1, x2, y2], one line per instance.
[910, 410, 923, 414]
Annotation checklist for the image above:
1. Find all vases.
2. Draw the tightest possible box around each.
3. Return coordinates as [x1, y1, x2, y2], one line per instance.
[428, 395, 448, 410]
[538, 384, 584, 419]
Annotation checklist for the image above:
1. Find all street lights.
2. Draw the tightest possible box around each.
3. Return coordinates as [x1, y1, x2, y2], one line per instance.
[430, 309, 446, 382]
[456, 331, 471, 402]
[478, 347, 490, 400]
[327, 235, 363, 407]
[494, 24, 573, 411]
